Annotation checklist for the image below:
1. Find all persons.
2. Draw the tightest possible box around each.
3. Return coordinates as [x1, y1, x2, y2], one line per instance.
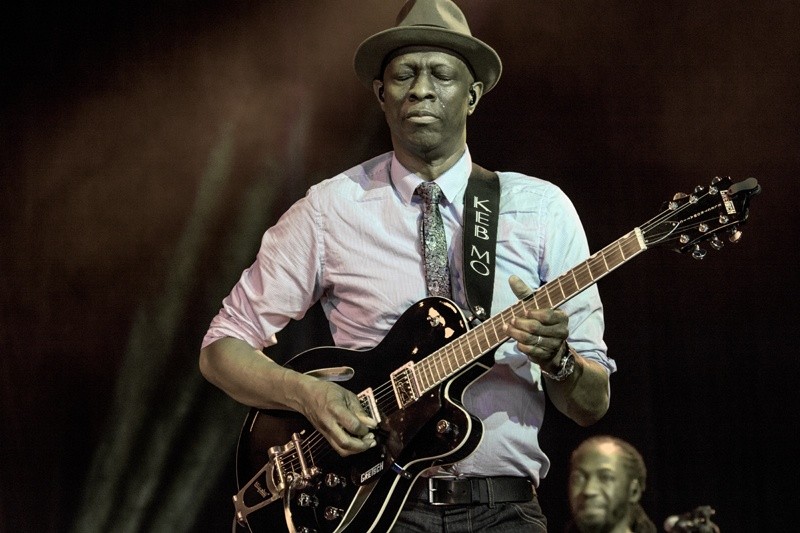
[197, 1, 614, 533]
[569, 435, 659, 533]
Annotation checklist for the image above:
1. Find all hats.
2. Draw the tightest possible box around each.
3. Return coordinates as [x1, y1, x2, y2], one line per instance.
[352, 0, 502, 95]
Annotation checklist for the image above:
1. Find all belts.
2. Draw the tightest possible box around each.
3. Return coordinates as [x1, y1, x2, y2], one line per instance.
[406, 474, 537, 507]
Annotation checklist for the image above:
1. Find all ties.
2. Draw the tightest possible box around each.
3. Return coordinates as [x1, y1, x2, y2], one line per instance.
[414, 182, 453, 302]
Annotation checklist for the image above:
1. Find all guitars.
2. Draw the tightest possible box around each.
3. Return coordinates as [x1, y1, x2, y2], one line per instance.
[232, 172, 761, 533]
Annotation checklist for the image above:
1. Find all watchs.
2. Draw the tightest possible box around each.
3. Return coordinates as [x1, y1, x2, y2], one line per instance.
[541, 344, 575, 382]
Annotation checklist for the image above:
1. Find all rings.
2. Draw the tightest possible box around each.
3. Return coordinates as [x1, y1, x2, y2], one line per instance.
[537, 336, 543, 345]
